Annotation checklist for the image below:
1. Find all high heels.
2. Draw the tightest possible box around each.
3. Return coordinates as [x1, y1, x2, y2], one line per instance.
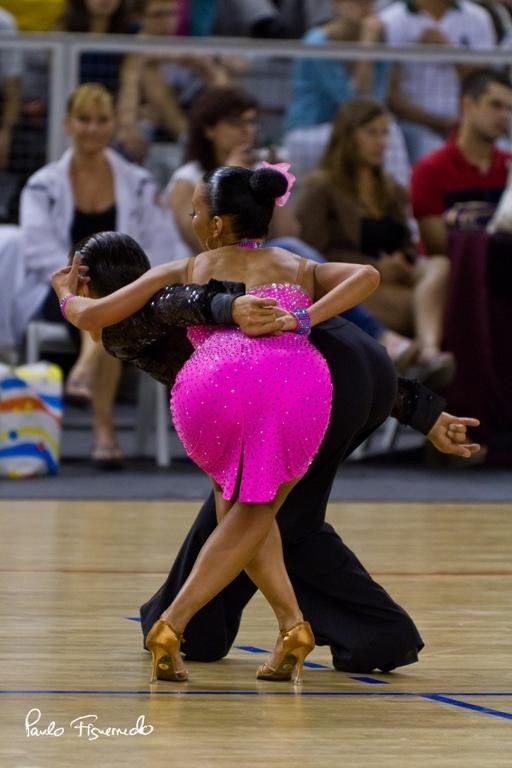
[147, 622, 189, 684]
[257, 621, 316, 680]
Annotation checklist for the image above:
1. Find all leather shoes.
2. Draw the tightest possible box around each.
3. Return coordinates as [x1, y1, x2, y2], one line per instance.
[328, 648, 420, 670]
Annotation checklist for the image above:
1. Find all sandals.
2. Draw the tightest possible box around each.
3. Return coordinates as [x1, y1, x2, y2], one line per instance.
[65, 376, 94, 409]
[93, 443, 124, 470]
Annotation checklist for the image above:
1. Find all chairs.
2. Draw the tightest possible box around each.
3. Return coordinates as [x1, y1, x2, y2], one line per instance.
[24, 313, 171, 470]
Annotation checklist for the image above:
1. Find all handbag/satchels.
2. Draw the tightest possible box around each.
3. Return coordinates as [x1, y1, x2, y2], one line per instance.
[0, 360, 62, 483]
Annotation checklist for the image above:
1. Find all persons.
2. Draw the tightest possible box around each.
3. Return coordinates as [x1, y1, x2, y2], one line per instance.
[284, 0, 392, 132]
[293, 95, 450, 380]
[119, 0, 226, 164]
[380, 0, 497, 164]
[49, 0, 144, 161]
[406, 67, 512, 255]
[46, 165, 380, 687]
[161, 87, 301, 255]
[0, 7, 32, 217]
[16, 82, 192, 471]
[74, 230, 487, 675]
